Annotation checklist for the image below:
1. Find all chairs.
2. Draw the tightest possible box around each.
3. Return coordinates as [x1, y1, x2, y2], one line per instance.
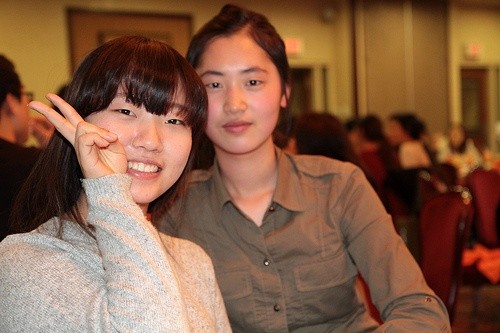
[379, 162, 500, 333]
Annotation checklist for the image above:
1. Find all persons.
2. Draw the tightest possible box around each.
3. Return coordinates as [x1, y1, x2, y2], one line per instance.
[0, 32, 233, 333]
[0, 53, 483, 259]
[154, 3, 453, 333]
